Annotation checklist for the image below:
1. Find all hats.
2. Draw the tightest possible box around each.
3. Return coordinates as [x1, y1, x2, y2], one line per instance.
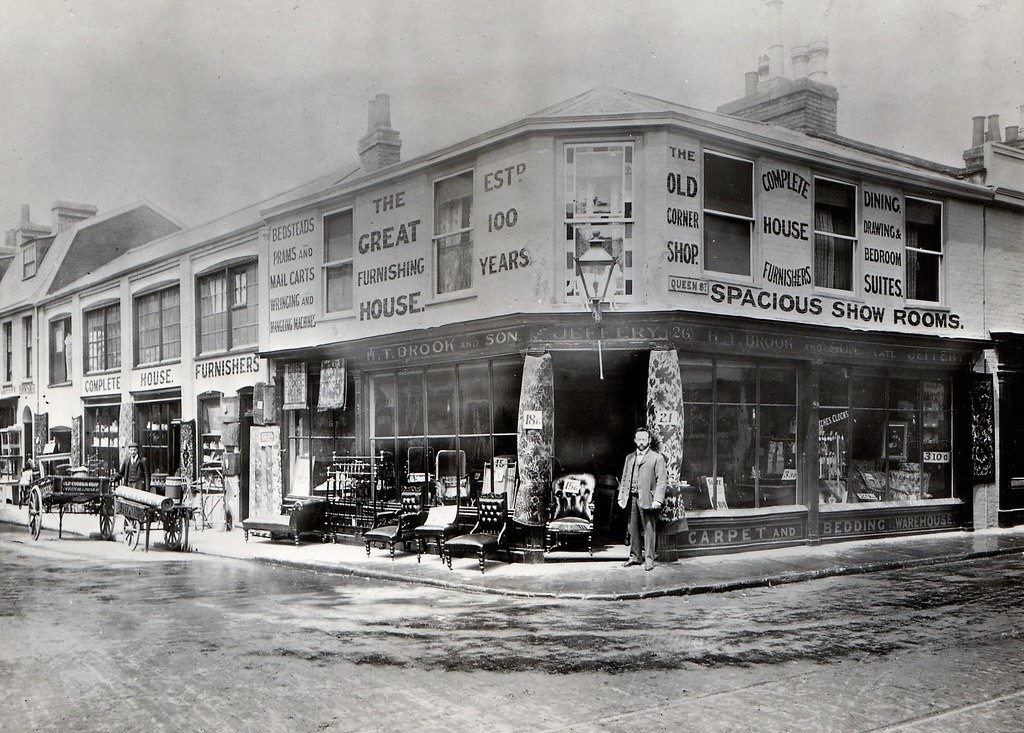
[128, 442, 139, 448]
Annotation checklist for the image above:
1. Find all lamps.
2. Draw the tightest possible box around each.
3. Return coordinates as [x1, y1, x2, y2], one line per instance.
[571, 229, 620, 327]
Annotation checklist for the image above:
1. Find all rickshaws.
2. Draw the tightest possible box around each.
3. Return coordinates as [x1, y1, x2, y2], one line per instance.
[25, 474, 197, 552]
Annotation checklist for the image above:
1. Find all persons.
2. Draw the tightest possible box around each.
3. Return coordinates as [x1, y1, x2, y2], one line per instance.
[109, 441, 151, 535]
[617, 426, 667, 571]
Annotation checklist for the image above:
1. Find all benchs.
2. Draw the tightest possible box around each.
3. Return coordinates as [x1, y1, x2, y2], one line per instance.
[243, 498, 328, 546]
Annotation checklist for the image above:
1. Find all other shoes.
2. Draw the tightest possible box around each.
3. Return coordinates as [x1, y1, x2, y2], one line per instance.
[645, 562, 653, 570]
[621, 559, 641, 566]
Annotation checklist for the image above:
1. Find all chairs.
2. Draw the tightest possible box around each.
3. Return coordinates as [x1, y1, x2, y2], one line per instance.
[361, 457, 594, 570]
